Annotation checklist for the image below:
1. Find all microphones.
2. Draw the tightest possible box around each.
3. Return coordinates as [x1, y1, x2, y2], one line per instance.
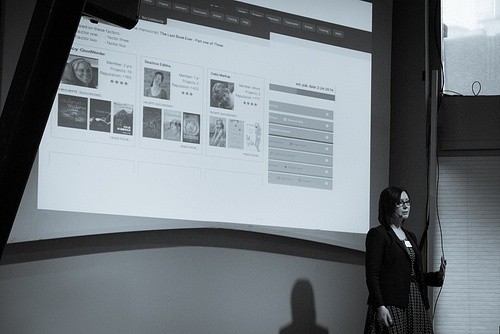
[394, 211, 402, 218]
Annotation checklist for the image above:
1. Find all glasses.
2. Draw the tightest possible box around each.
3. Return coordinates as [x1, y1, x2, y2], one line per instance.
[394, 200, 410, 208]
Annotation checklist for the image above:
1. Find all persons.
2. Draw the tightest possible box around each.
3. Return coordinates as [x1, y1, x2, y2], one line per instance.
[63, 58, 96, 87]
[211, 82, 235, 109]
[145, 72, 167, 98]
[364, 187, 447, 334]
[212, 117, 225, 146]
